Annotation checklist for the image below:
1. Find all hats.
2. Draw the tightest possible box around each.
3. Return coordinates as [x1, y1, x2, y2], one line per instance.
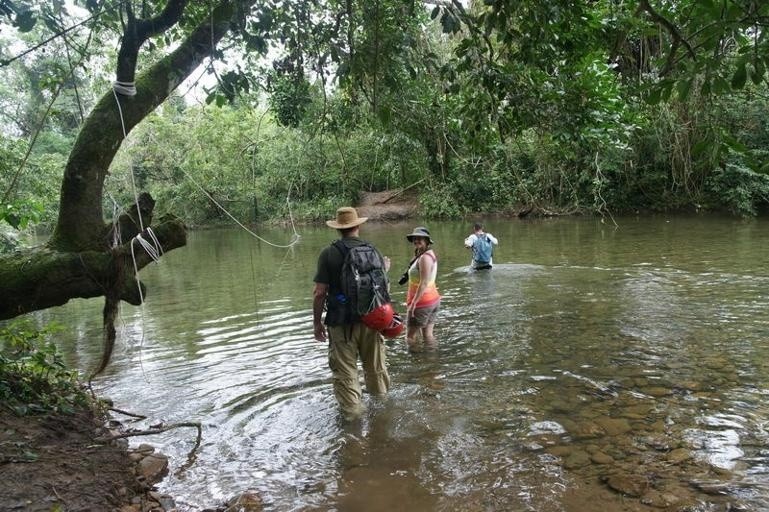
[326, 206, 368, 230]
[406, 227, 434, 245]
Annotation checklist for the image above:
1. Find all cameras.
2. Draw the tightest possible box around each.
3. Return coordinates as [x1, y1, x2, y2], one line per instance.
[398, 272, 409, 286]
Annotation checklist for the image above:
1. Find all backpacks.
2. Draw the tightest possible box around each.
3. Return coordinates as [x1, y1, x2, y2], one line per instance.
[331, 238, 391, 324]
[471, 232, 493, 264]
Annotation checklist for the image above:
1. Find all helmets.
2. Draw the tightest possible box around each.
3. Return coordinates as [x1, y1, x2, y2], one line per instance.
[382, 312, 403, 338]
[363, 302, 394, 333]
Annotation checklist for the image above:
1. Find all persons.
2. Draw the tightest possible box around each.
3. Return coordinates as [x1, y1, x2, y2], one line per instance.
[337, 405, 438, 512]
[404, 226, 441, 348]
[463, 222, 500, 269]
[409, 342, 444, 399]
[312, 206, 393, 421]
